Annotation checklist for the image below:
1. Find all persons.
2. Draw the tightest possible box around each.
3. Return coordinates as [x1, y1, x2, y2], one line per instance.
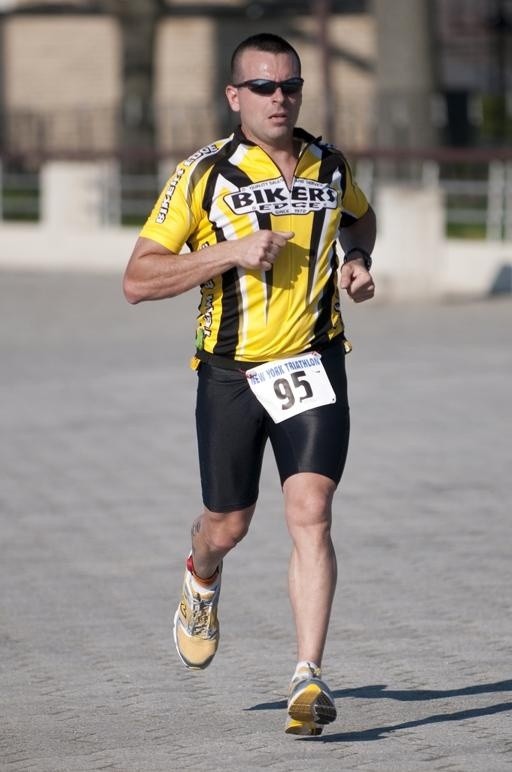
[122, 34, 379, 738]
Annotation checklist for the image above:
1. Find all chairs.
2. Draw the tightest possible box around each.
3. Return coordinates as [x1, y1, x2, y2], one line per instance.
[174, 551, 222, 670]
[285, 661, 337, 736]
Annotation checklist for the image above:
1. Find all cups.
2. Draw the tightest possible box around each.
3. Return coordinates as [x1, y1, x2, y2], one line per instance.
[233, 78, 305, 96]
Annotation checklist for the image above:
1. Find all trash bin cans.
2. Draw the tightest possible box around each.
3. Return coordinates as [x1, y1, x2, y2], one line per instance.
[344, 246, 374, 271]
